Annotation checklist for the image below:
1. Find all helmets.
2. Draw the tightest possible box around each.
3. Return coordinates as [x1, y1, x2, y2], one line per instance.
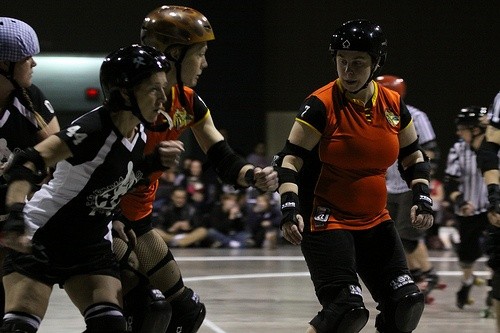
[328, 19, 388, 65]
[455, 105, 488, 127]
[0, 17, 40, 63]
[375, 75, 407, 97]
[140, 5, 215, 54]
[99, 44, 172, 105]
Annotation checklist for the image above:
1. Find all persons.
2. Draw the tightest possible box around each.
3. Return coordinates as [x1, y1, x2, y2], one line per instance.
[0, 41, 171, 333]
[0, 17, 62, 228]
[424, 177, 461, 250]
[272, 19, 436, 333]
[111, 5, 278, 333]
[374, 74, 448, 303]
[444, 92, 500, 333]
[150, 141, 289, 249]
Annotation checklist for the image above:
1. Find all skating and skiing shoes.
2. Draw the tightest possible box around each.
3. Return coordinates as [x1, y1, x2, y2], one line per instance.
[410, 266, 447, 304]
[455, 274, 484, 309]
[480, 291, 498, 317]
